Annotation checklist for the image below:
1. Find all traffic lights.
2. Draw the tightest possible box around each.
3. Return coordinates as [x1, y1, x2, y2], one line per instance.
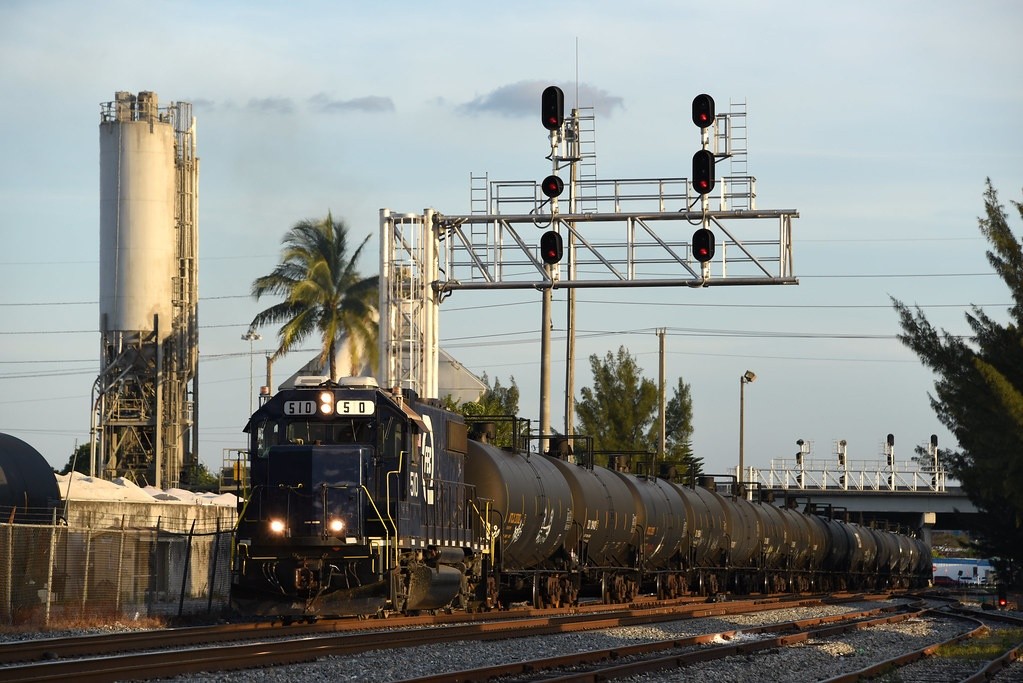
[692, 94, 715, 129]
[796, 454, 801, 464]
[540, 231, 563, 264]
[796, 475, 801, 482]
[840, 476, 845, 484]
[692, 229, 715, 262]
[692, 149, 715, 195]
[999, 595, 1007, 608]
[542, 176, 564, 198]
[839, 453, 844, 465]
[541, 86, 564, 132]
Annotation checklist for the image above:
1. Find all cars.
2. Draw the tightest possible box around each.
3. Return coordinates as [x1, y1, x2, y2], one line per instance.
[934, 576, 958, 585]
[958, 577, 975, 585]
[975, 577, 988, 585]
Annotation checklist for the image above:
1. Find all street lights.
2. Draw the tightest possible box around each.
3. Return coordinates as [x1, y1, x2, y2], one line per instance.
[739, 371, 757, 483]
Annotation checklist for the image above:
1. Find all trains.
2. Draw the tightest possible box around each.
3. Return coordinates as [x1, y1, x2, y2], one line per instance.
[232, 376, 935, 617]
